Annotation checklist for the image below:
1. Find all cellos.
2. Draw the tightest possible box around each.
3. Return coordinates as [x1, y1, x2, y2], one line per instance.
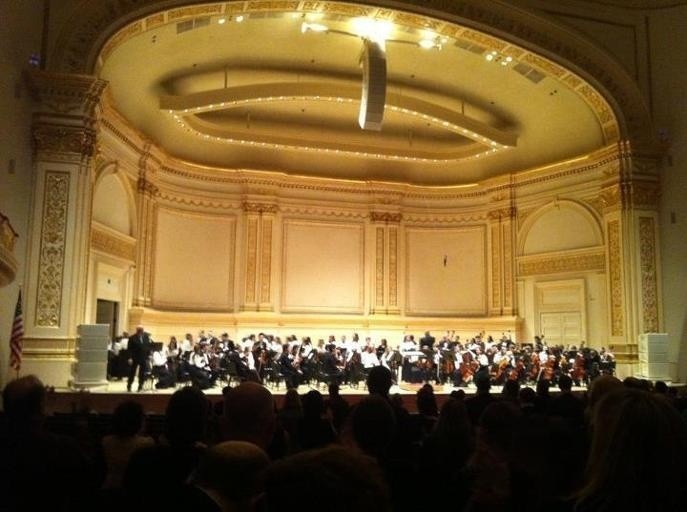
[423, 335, 585, 384]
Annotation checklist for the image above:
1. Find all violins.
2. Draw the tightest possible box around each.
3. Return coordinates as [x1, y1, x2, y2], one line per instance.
[257, 339, 345, 372]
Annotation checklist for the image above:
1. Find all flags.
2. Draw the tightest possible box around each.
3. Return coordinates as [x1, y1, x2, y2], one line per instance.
[9, 289, 24, 371]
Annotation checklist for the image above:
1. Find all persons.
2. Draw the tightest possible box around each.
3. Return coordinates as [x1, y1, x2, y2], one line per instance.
[106, 326, 616, 392]
[1, 363, 686, 512]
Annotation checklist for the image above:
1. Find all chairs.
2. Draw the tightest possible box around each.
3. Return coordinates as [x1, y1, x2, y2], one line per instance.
[108, 330, 619, 390]
